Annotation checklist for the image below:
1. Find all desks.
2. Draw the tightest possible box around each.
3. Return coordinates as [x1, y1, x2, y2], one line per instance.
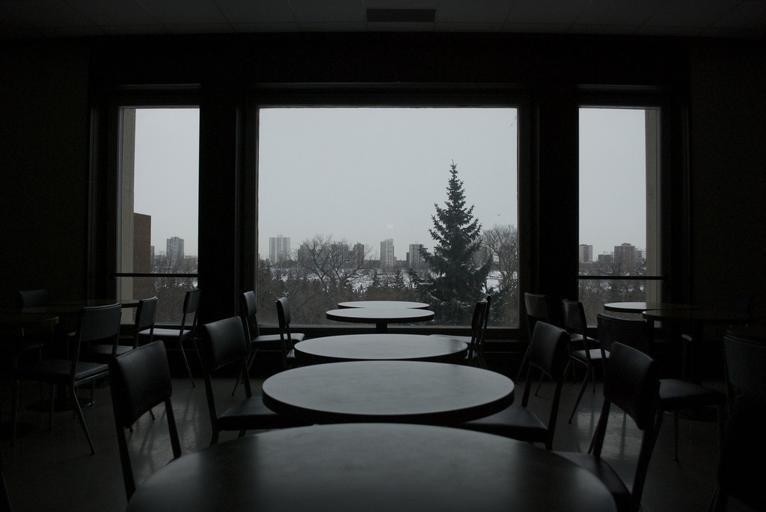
[604, 300, 672, 356]
[258, 296, 520, 421]
[643, 309, 741, 383]
[3, 311, 60, 418]
[122, 420, 620, 510]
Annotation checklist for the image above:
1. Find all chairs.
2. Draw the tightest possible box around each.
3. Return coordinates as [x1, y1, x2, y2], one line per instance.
[276, 298, 306, 368]
[13, 301, 121, 454]
[596, 313, 726, 477]
[559, 299, 619, 425]
[523, 320, 572, 447]
[193, 315, 297, 450]
[232, 290, 295, 395]
[138, 290, 203, 387]
[589, 341, 664, 511]
[108, 341, 183, 499]
[526, 293, 597, 396]
[88, 295, 160, 407]
